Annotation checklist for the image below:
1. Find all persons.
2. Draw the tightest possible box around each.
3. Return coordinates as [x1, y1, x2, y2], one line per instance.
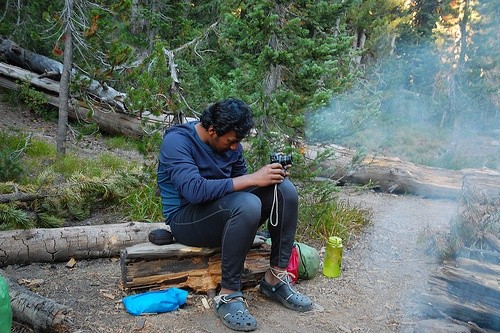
[158, 99, 313, 331]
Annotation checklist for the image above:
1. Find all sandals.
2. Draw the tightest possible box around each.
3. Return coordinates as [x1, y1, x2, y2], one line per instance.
[259, 277, 314, 312]
[214, 291, 257, 331]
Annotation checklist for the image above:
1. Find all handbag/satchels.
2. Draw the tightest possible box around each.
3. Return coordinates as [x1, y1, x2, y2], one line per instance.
[148, 228, 174, 245]
[266, 238, 320, 286]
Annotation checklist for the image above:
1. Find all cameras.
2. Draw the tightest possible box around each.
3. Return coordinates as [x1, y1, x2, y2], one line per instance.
[269, 152, 294, 178]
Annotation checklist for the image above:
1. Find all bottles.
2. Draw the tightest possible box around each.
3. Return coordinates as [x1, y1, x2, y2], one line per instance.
[322, 236, 342, 278]
